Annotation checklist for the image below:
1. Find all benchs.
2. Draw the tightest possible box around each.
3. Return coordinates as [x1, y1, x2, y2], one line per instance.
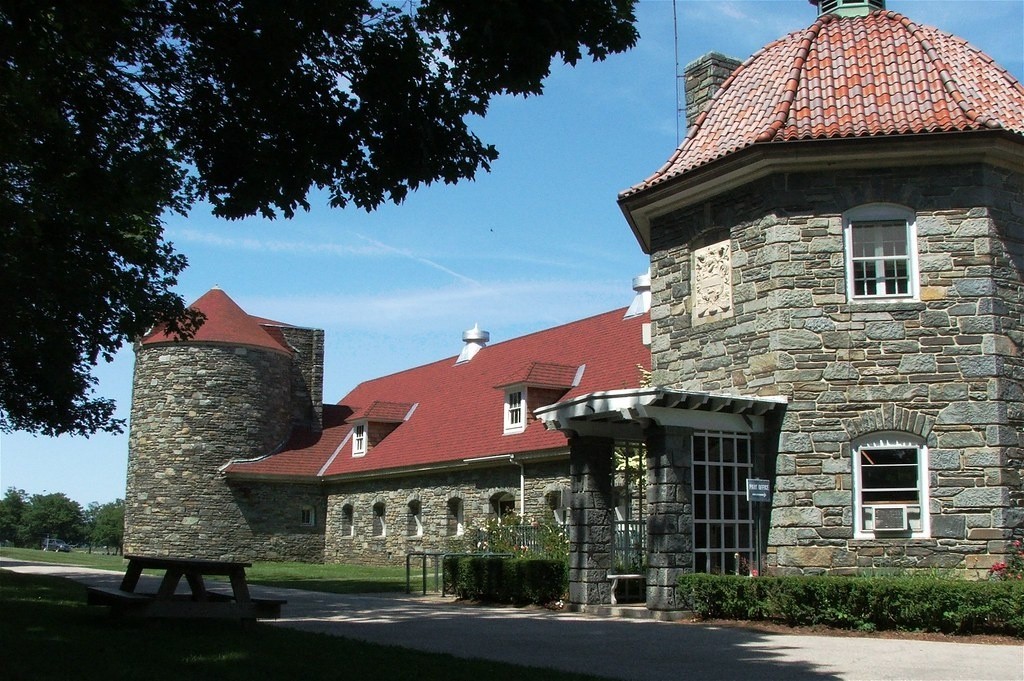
[86, 586, 287, 632]
[606, 574, 647, 607]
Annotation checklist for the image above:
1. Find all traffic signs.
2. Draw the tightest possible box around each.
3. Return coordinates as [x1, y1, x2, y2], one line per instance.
[745, 478, 772, 503]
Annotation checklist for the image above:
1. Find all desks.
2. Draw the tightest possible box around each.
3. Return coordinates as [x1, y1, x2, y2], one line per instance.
[108, 555, 259, 632]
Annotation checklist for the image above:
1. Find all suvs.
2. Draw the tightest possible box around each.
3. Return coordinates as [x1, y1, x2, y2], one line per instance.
[41, 538, 72, 552]
